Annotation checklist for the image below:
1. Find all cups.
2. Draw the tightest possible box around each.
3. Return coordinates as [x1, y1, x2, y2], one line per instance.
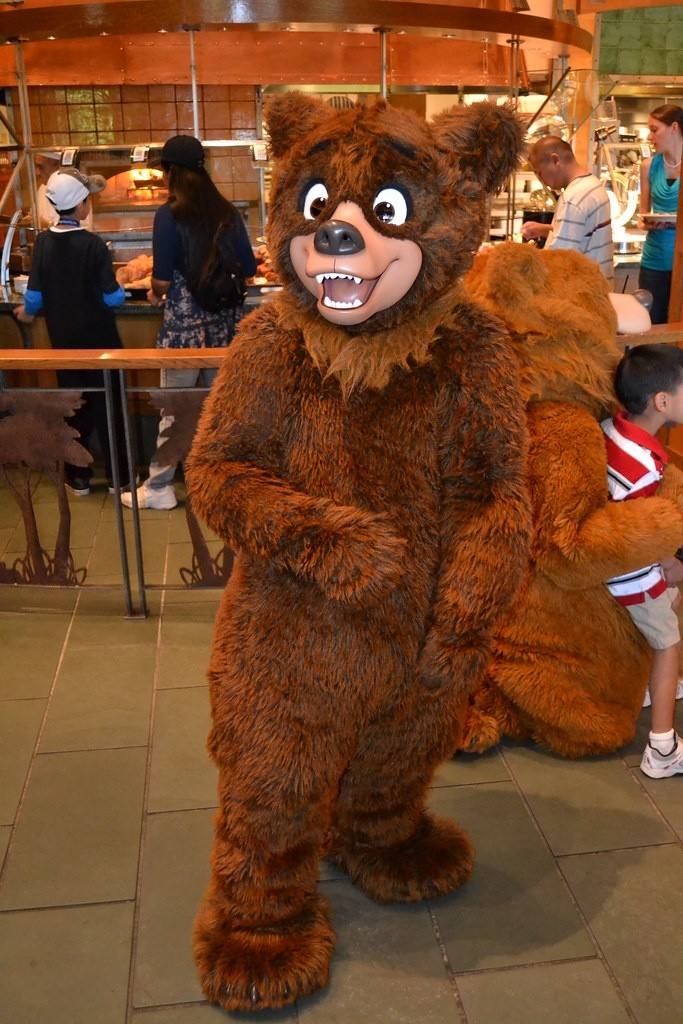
[14, 277, 29, 294]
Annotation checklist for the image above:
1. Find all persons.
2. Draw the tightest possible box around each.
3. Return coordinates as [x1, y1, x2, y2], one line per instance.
[519, 136, 614, 292]
[636, 105, 683, 326]
[119, 135, 258, 510]
[13, 166, 140, 497]
[598, 343, 683, 778]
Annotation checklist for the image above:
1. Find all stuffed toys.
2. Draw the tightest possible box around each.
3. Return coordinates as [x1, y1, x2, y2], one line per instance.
[185, 89, 538, 1014]
[453, 242, 683, 767]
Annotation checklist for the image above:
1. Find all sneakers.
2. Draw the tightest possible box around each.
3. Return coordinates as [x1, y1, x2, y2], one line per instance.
[642, 678, 683, 707]
[640, 731, 683, 778]
[120, 483, 178, 510]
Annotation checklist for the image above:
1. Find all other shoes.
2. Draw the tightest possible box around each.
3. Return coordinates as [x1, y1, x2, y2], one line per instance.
[108, 472, 139, 493]
[63, 480, 90, 496]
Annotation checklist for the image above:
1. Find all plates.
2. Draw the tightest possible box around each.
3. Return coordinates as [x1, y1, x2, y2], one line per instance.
[638, 213, 678, 222]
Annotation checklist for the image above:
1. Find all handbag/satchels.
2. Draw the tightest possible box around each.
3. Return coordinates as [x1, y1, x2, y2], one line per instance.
[192, 204, 246, 311]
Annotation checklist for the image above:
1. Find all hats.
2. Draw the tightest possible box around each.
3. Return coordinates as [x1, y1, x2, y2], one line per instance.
[147, 135, 206, 167]
[44, 167, 106, 210]
